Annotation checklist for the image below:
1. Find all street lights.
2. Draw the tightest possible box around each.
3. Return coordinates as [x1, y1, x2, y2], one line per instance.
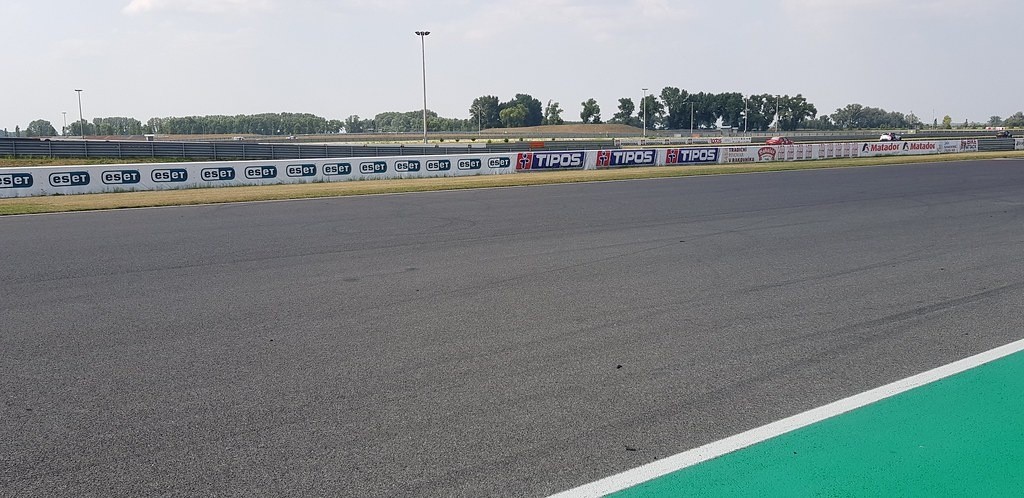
[75, 89, 84, 140]
[641, 88, 647, 138]
[62, 111, 68, 137]
[690, 101, 694, 135]
[775, 94, 780, 133]
[477, 107, 482, 135]
[415, 31, 430, 144]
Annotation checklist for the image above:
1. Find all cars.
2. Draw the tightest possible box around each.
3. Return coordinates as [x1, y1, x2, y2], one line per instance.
[878, 133, 902, 141]
[995, 130, 1014, 138]
[766, 136, 795, 146]
